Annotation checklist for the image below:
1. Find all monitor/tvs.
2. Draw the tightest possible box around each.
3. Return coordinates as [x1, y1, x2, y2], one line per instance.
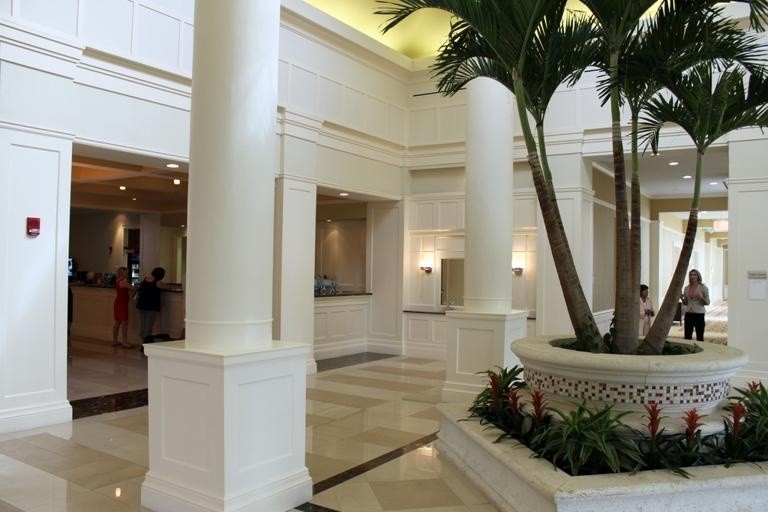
[68, 256, 75, 283]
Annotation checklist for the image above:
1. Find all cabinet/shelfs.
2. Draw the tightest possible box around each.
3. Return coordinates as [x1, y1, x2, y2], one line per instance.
[673, 302, 686, 326]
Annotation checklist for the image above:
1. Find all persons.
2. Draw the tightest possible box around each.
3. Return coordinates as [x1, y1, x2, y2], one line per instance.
[679, 268, 710, 341]
[69, 286, 74, 360]
[111, 267, 136, 348]
[134, 267, 167, 350]
[639, 284, 654, 336]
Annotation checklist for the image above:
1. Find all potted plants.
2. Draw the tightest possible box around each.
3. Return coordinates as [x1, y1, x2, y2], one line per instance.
[374, 0, 768, 454]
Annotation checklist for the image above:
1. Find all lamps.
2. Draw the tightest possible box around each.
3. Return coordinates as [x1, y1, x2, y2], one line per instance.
[510, 257, 524, 273]
[419, 260, 432, 273]
